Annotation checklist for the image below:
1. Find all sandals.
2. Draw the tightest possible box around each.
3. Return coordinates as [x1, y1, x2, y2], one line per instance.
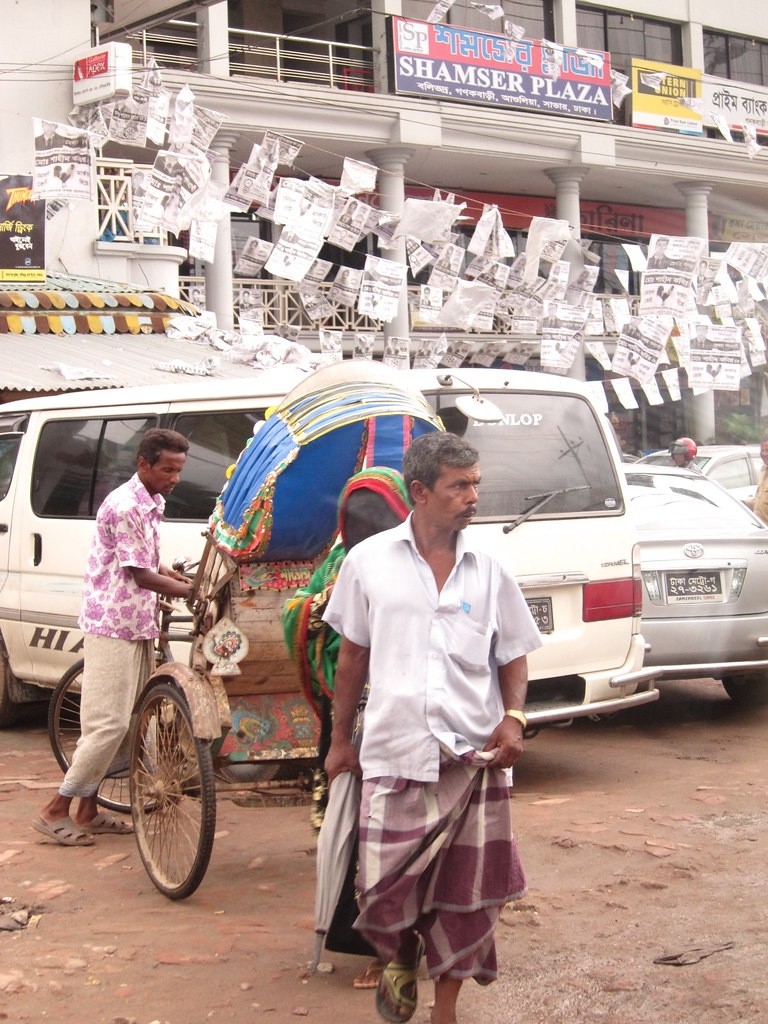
[375, 937, 424, 1021]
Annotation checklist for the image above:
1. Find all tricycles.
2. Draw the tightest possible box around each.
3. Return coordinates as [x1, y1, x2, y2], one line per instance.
[48, 352, 471, 901]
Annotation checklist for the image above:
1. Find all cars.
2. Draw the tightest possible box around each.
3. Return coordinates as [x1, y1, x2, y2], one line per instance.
[619, 464, 768, 708]
[628, 443, 768, 521]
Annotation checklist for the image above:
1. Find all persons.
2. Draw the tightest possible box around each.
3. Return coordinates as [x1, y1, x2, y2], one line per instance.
[319, 430, 545, 1022]
[741, 436, 768, 528]
[668, 437, 702, 474]
[30, 428, 193, 847]
[281, 467, 420, 832]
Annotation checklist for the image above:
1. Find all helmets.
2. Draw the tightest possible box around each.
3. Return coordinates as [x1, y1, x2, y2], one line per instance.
[668, 438, 697, 460]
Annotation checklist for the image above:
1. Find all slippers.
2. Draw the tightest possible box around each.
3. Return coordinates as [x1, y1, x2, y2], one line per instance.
[353, 953, 387, 987]
[32, 815, 95, 846]
[77, 811, 136, 834]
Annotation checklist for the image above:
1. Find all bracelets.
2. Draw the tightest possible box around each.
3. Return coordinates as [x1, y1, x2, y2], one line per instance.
[503, 710, 527, 729]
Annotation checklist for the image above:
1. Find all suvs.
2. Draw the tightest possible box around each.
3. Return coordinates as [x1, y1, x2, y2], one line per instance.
[4, 365, 664, 786]
